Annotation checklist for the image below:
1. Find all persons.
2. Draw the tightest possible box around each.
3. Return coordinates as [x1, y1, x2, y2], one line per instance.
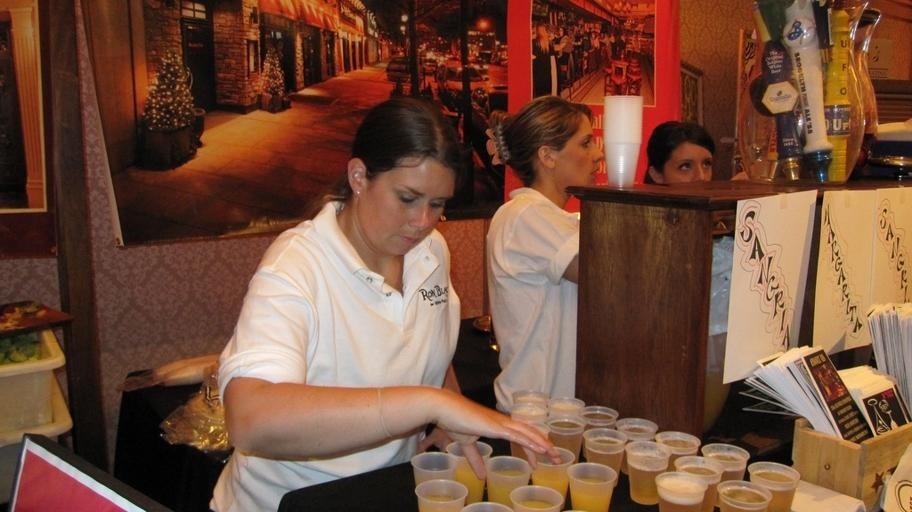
[206, 93, 564, 512]
[468, 95, 607, 417]
[640, 119, 767, 188]
[533, 18, 627, 88]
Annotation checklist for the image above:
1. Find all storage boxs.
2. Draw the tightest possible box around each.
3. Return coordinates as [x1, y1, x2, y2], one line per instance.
[1, 329, 67, 435]
[1, 370, 77, 505]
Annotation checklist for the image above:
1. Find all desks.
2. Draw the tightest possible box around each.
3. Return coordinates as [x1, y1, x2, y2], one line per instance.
[279, 433, 871, 512]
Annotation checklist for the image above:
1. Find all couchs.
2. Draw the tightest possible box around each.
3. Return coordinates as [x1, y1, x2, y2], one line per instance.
[114, 305, 503, 512]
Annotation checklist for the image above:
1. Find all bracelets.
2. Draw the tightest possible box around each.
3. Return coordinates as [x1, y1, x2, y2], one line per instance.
[378, 385, 396, 441]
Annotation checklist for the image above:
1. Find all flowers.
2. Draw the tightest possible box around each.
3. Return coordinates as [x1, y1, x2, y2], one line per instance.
[133, 45, 197, 131]
[259, 40, 287, 96]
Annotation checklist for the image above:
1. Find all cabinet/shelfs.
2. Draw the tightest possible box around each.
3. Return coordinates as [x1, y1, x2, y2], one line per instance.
[569, 172, 910, 447]
[0, 2, 111, 511]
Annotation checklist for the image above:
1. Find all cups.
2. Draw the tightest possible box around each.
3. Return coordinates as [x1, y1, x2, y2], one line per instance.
[416, 478, 467, 512]
[543, 409, 584, 458]
[583, 424, 626, 483]
[616, 415, 659, 445]
[674, 454, 720, 511]
[547, 395, 586, 418]
[505, 391, 548, 454]
[699, 441, 750, 487]
[626, 440, 668, 505]
[484, 453, 531, 506]
[578, 404, 620, 430]
[654, 471, 708, 512]
[412, 452, 451, 496]
[716, 480, 771, 510]
[462, 501, 512, 510]
[748, 459, 799, 510]
[509, 485, 563, 510]
[654, 428, 701, 470]
[869, 123, 911, 183]
[602, 95, 644, 190]
[530, 444, 577, 506]
[564, 462, 619, 511]
[445, 439, 492, 502]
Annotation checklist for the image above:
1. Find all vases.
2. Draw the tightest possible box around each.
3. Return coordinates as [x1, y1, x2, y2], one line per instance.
[135, 124, 199, 170]
[261, 91, 287, 114]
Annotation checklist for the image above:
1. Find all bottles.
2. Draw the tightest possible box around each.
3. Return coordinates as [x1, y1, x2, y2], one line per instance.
[735, 1, 884, 182]
